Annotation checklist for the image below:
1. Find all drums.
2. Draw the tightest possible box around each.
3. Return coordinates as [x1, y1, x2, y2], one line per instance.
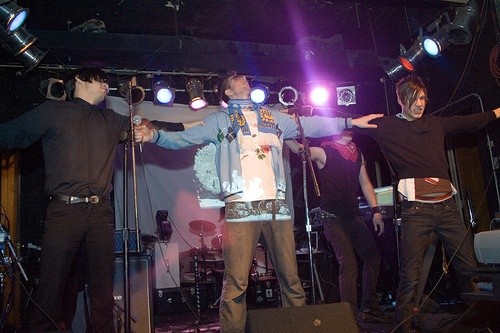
[252, 242, 274, 276]
[211, 235, 223, 253]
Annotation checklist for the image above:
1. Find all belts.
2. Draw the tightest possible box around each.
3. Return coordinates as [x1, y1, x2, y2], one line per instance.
[56, 193, 111, 205]
[225, 199, 292, 219]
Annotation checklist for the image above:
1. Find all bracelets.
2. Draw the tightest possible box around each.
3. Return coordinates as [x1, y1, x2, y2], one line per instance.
[371, 206, 382, 213]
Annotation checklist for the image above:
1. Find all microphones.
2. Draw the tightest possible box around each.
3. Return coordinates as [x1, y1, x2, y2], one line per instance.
[133, 114, 143, 153]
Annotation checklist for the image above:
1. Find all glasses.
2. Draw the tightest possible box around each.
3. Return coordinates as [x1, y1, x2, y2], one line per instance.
[91, 73, 108, 83]
[231, 74, 247, 87]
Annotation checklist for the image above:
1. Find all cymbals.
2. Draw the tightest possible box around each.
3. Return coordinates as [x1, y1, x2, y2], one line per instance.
[190, 220, 217, 231]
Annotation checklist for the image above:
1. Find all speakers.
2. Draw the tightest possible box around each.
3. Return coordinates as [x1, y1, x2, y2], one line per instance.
[71, 256, 154, 333]
[245, 301, 360, 333]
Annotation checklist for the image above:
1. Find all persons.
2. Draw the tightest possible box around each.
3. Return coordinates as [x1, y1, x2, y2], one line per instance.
[279, 106, 385, 323]
[276, 74, 500, 333]
[0, 66, 205, 333]
[132, 73, 384, 333]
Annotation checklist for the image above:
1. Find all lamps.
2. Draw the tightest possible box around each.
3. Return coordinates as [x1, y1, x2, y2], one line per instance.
[116, 66, 357, 111]
[0, 0, 69, 102]
[385, 0, 479, 82]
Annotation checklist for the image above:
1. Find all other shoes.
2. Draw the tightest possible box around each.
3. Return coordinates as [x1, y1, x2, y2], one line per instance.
[361, 307, 393, 322]
